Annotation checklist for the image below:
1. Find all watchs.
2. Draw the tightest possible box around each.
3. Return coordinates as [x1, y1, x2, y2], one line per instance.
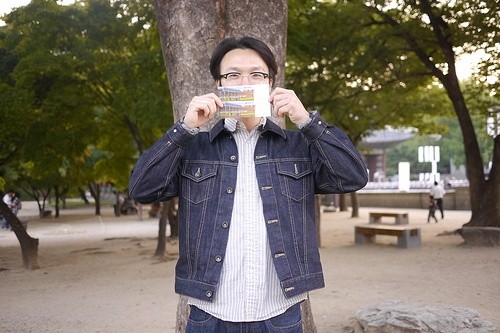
[179, 116, 201, 136]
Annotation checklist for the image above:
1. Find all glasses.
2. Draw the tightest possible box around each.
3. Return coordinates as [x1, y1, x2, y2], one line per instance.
[218, 72, 272, 83]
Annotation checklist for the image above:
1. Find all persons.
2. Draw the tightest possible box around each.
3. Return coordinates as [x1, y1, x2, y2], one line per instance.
[129, 36, 368, 333]
[432, 179, 446, 219]
[2, 189, 13, 230]
[427, 194, 438, 223]
[7, 191, 20, 230]
[109, 187, 140, 216]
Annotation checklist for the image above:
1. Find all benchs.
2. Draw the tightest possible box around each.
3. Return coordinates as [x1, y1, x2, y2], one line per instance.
[369, 212, 409, 224]
[354, 225, 422, 249]
